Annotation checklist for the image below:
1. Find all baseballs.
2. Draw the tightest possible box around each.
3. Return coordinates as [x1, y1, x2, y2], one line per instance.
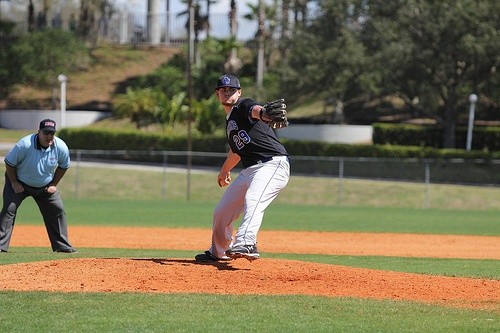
[220, 180, 225, 185]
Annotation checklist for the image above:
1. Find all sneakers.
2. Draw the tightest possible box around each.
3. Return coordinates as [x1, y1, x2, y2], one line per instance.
[226, 243, 260, 261]
[194, 251, 234, 261]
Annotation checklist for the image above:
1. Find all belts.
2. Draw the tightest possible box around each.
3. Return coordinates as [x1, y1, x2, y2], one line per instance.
[246, 156, 291, 167]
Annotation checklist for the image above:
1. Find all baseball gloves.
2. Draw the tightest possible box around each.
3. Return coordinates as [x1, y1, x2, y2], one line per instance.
[260, 98, 289, 129]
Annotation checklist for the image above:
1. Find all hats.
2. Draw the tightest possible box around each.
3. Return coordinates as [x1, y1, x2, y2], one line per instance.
[39, 119, 55, 132]
[215, 74, 241, 89]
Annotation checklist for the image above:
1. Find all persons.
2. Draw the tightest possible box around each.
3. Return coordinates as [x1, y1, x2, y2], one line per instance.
[0, 119, 78, 253]
[195, 74, 290, 265]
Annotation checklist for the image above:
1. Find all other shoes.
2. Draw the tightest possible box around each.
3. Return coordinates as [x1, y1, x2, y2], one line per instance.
[56, 246, 76, 254]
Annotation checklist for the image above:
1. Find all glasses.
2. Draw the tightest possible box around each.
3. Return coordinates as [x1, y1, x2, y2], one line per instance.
[42, 131, 55, 135]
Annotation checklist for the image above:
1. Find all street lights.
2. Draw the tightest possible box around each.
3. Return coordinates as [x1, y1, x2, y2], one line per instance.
[57, 74, 68, 129]
[466, 93, 478, 151]
